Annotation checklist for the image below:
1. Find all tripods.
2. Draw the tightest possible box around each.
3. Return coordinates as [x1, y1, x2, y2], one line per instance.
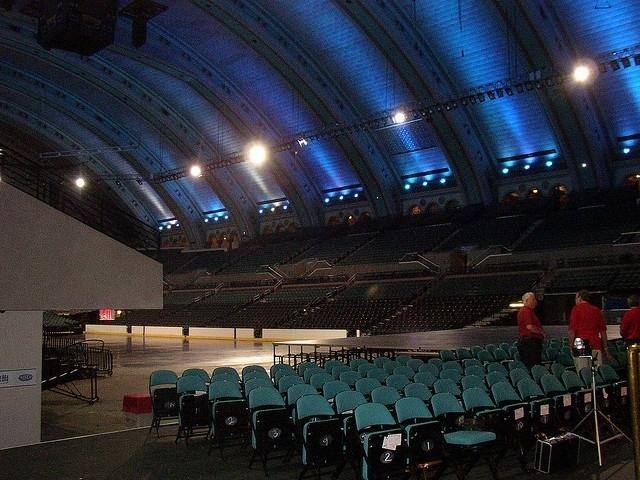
[568, 374, 633, 467]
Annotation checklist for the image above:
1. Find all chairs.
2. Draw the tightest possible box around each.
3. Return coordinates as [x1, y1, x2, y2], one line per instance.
[42, 347, 101, 408]
[44, 311, 171, 331]
[165, 205, 640, 277]
[164, 277, 639, 332]
[149, 336, 639, 480]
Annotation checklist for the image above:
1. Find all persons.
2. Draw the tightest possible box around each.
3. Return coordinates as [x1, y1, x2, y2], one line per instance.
[518, 291, 550, 375]
[620, 294, 640, 353]
[567, 290, 614, 376]
